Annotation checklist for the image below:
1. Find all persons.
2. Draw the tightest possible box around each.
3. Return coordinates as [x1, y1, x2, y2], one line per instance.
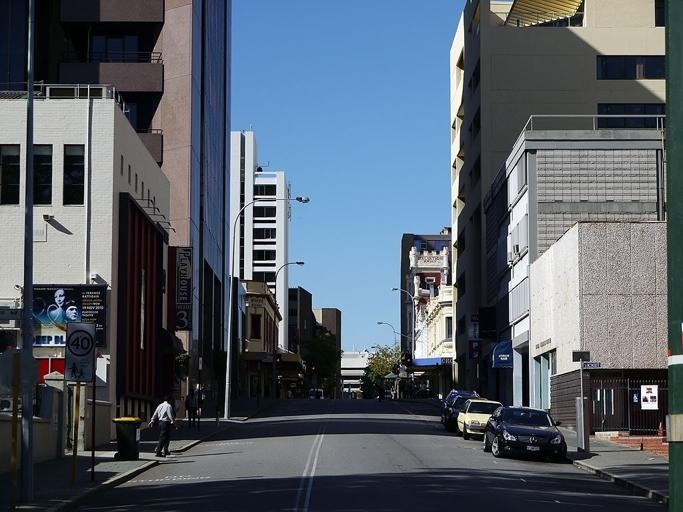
[147, 394, 175, 457]
[63, 300, 78, 321]
[184, 388, 197, 423]
[49, 289, 66, 316]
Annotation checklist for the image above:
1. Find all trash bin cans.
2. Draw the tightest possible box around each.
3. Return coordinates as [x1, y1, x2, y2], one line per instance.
[112, 417, 142, 461]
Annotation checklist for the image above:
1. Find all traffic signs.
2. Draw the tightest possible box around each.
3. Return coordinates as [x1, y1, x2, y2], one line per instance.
[62, 321, 97, 382]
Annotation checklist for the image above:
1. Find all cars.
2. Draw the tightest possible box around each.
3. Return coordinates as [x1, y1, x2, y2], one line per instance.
[440, 388, 567, 463]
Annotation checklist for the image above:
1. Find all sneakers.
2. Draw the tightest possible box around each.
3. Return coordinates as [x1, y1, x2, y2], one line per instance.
[154, 451, 170, 457]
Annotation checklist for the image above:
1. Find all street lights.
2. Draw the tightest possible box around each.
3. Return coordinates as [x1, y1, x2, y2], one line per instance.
[374, 285, 424, 412]
[223, 188, 314, 438]
